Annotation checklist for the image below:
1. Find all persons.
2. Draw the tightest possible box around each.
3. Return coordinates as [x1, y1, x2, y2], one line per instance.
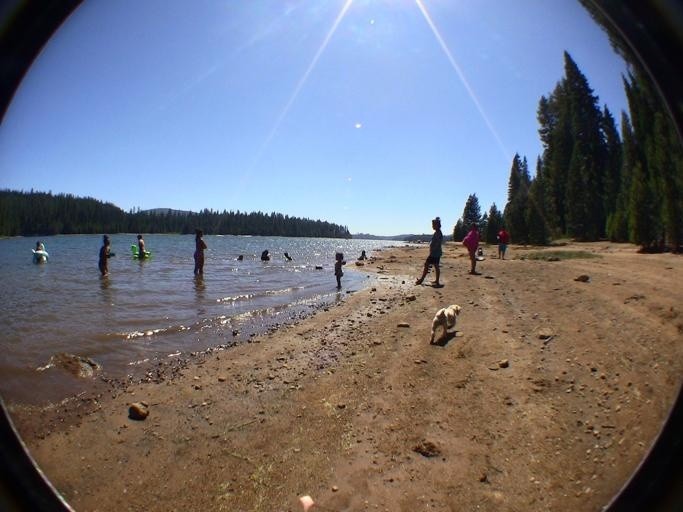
[137, 235, 146, 261]
[283, 253, 292, 261]
[237, 255, 243, 260]
[496, 225, 510, 260]
[462, 222, 480, 274]
[415, 217, 443, 287]
[260, 250, 270, 261]
[334, 253, 346, 289]
[193, 228, 207, 273]
[356, 250, 367, 260]
[31, 240, 49, 265]
[98, 234, 115, 277]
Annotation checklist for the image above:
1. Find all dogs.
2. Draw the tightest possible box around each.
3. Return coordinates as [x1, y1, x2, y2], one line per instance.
[430, 304, 463, 344]
